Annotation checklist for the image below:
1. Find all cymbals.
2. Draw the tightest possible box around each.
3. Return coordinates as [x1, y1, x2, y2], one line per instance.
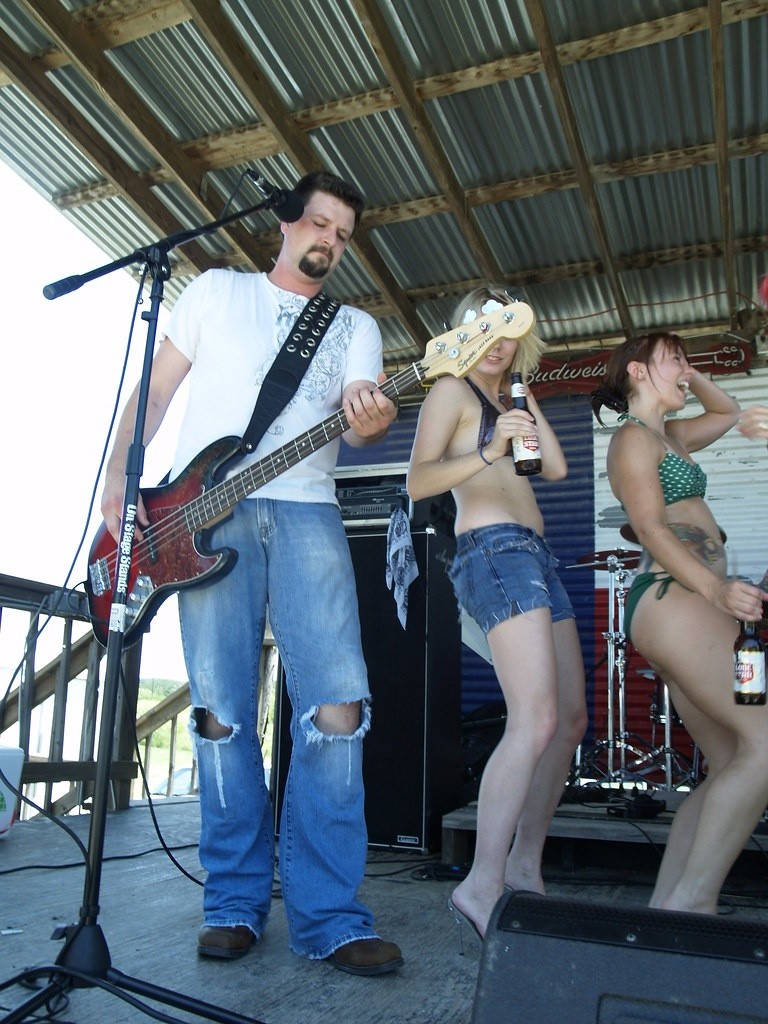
[577, 550, 642, 570]
[619, 522, 727, 545]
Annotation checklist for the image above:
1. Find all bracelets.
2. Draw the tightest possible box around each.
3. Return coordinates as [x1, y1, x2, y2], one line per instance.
[480, 447, 493, 465]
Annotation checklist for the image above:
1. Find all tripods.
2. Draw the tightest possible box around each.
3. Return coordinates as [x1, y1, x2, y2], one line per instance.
[0, 200, 263, 1024]
[564, 554, 711, 802]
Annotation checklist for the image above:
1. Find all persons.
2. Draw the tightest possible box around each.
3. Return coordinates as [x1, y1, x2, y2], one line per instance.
[593, 273, 768, 915]
[101, 173, 405, 977]
[406, 284, 588, 956]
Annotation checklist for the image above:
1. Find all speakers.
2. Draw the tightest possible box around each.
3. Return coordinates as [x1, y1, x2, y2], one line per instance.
[267, 524, 461, 855]
[472, 890, 768, 1024]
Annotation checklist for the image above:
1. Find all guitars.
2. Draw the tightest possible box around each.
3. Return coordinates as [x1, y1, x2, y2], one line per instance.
[86, 300, 535, 648]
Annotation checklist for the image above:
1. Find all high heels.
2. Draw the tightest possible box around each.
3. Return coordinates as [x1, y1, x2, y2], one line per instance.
[448, 896, 486, 957]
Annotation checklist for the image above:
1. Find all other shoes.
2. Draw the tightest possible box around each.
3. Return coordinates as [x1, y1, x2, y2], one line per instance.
[196, 923, 257, 959]
[330, 936, 404, 977]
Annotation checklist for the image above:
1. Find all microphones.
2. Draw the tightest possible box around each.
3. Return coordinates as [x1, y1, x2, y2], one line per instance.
[249, 168, 305, 223]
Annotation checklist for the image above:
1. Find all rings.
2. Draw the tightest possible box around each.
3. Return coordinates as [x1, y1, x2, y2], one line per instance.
[761, 423, 768, 430]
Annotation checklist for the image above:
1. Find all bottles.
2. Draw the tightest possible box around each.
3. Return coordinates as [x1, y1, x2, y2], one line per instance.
[733, 619, 767, 705]
[510, 371, 542, 475]
